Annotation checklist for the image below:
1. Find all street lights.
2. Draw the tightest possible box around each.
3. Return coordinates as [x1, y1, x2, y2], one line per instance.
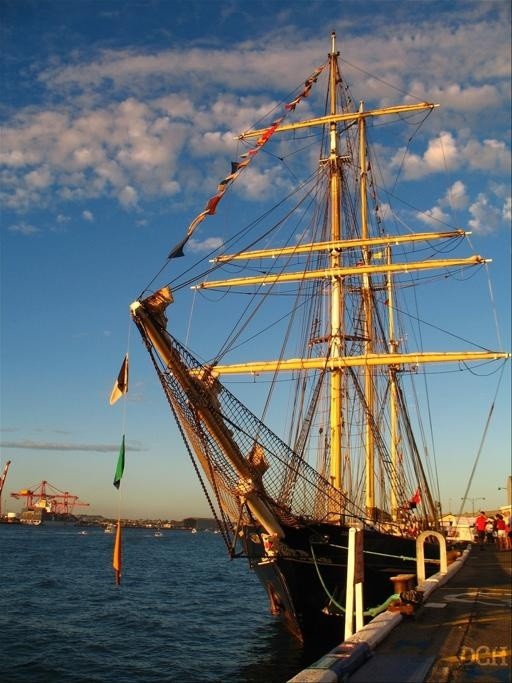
[459, 496, 486, 516]
[497, 486, 508, 492]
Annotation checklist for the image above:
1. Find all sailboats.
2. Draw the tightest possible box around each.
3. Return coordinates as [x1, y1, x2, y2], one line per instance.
[121, 27, 512, 653]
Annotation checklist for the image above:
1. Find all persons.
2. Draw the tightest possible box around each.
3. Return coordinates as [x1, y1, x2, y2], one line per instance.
[405, 511, 512, 552]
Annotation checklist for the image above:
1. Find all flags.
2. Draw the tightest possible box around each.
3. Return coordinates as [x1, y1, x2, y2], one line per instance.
[113, 434, 125, 491]
[111, 519, 123, 587]
[108, 354, 129, 406]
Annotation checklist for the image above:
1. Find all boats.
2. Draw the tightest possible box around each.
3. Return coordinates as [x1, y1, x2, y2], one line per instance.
[191, 527, 197, 533]
[103, 523, 115, 534]
[153, 530, 161, 537]
[77, 530, 87, 534]
